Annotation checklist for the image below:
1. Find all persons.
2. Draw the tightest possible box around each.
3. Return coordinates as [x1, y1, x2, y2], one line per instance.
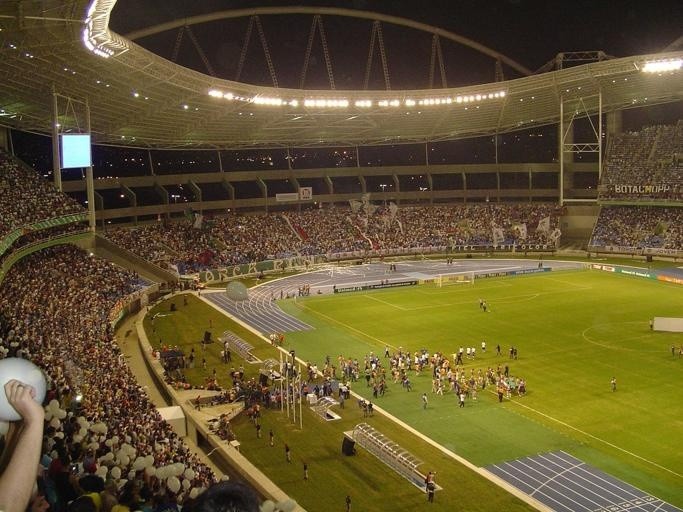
[678, 348, 681, 357]
[479, 297, 483, 309]
[0, 144, 264, 512]
[345, 495, 352, 512]
[591, 117, 682, 254]
[264, 343, 525, 419]
[268, 429, 274, 441]
[106, 196, 567, 279]
[284, 443, 291, 464]
[610, 376, 616, 392]
[425, 471, 432, 491]
[303, 463, 307, 480]
[269, 332, 282, 347]
[482, 301, 486, 312]
[426, 482, 435, 502]
[671, 346, 674, 355]
[649, 318, 652, 329]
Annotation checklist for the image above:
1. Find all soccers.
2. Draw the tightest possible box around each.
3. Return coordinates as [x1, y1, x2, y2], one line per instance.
[0, 357, 47, 422]
